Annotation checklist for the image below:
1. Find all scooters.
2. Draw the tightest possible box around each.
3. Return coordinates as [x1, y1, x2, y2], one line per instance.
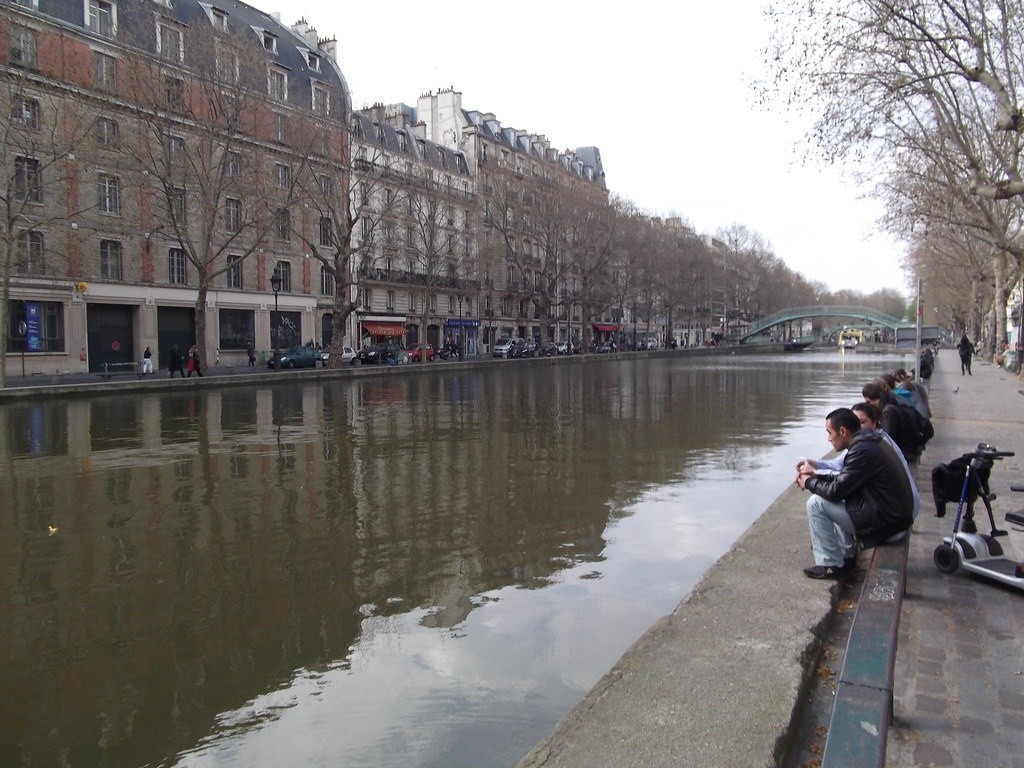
[933, 450, 1024, 591]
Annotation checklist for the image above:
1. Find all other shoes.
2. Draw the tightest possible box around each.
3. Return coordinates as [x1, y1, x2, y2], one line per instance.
[803, 564, 849, 580]
[844, 555, 858, 567]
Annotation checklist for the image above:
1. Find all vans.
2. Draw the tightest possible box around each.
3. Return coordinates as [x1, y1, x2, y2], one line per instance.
[492, 338, 525, 358]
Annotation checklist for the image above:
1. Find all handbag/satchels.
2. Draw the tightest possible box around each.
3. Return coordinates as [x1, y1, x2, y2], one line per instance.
[187, 357, 195, 371]
[251, 356, 256, 361]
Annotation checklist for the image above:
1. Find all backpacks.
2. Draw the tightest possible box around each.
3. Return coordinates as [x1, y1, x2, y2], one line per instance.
[887, 399, 935, 440]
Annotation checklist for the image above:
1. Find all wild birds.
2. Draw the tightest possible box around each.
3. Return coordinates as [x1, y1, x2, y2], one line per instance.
[952, 386, 959, 394]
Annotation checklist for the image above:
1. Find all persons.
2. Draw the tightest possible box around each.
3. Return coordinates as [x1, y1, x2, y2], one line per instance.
[310, 337, 332, 351]
[187, 345, 204, 377]
[168, 344, 185, 378]
[796, 402, 921, 524]
[450, 341, 457, 358]
[908, 348, 935, 379]
[880, 373, 928, 418]
[862, 383, 925, 464]
[246, 340, 255, 367]
[891, 369, 933, 418]
[272, 345, 283, 371]
[994, 336, 1024, 374]
[142, 347, 155, 373]
[871, 377, 935, 442]
[796, 408, 915, 580]
[956, 333, 977, 375]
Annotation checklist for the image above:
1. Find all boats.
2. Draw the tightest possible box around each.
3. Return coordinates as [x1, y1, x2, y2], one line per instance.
[837, 329, 869, 350]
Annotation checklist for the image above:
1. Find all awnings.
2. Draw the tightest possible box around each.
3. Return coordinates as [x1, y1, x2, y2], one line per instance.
[592, 323, 619, 332]
[363, 321, 408, 336]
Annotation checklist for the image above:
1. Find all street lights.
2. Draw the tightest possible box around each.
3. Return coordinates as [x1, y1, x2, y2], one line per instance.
[457, 294, 465, 361]
[269, 274, 283, 372]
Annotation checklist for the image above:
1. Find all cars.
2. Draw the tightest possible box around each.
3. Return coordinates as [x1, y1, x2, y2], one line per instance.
[401, 343, 435, 362]
[572, 343, 601, 353]
[356, 343, 401, 365]
[598, 342, 617, 353]
[636, 338, 658, 351]
[511, 341, 539, 357]
[319, 346, 357, 366]
[554, 341, 574, 354]
[267, 346, 322, 369]
